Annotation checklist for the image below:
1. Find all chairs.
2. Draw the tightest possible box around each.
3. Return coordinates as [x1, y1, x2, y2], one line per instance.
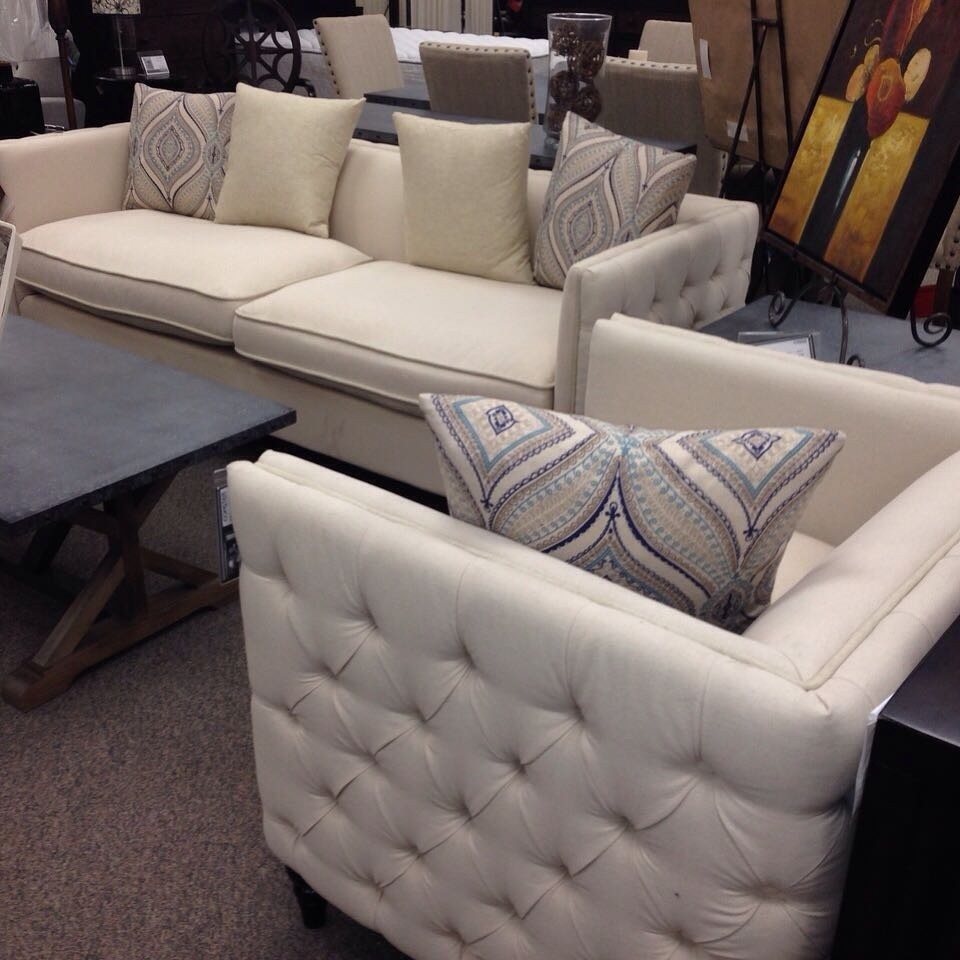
[638, 19, 696, 64]
[313, 13, 403, 99]
[418, 41, 538, 121]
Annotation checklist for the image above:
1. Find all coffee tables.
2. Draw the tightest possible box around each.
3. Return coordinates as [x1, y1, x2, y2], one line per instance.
[0, 302, 296, 713]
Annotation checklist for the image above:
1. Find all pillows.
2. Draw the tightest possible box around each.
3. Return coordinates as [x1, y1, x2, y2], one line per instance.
[215, 79, 363, 238]
[533, 108, 693, 289]
[125, 81, 236, 219]
[417, 394, 844, 634]
[392, 111, 532, 286]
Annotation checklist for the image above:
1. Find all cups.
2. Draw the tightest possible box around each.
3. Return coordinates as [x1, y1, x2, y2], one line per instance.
[628, 50, 648, 63]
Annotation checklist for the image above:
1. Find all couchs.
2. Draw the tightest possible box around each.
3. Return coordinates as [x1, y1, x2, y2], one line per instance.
[0, 122, 760, 497]
[251, 317, 960, 960]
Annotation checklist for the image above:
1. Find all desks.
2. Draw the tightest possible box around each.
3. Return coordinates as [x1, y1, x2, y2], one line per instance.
[364, 56, 700, 171]
